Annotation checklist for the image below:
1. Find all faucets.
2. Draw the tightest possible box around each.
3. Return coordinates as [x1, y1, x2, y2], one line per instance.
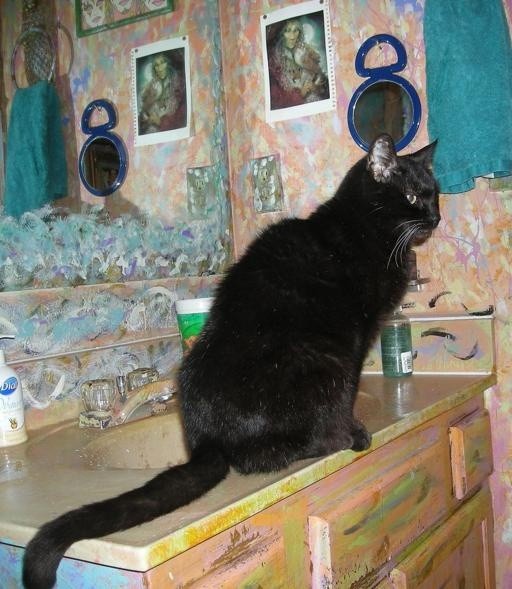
[114, 379, 176, 429]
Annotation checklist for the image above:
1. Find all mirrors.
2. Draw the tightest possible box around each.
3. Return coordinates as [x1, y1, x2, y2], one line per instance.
[0, 0, 234, 294]
[77, 100, 127, 196]
[347, 31, 425, 155]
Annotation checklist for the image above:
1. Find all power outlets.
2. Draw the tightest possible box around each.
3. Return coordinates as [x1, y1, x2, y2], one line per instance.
[248, 153, 283, 215]
[187, 167, 217, 224]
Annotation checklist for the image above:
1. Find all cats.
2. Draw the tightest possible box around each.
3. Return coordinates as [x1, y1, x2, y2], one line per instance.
[1, 132, 440, 588]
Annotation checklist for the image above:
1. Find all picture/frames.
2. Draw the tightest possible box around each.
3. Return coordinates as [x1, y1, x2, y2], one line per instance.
[76, 0, 173, 41]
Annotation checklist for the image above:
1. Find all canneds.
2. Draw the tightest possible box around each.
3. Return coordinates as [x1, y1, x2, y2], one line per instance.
[380, 306, 413, 379]
